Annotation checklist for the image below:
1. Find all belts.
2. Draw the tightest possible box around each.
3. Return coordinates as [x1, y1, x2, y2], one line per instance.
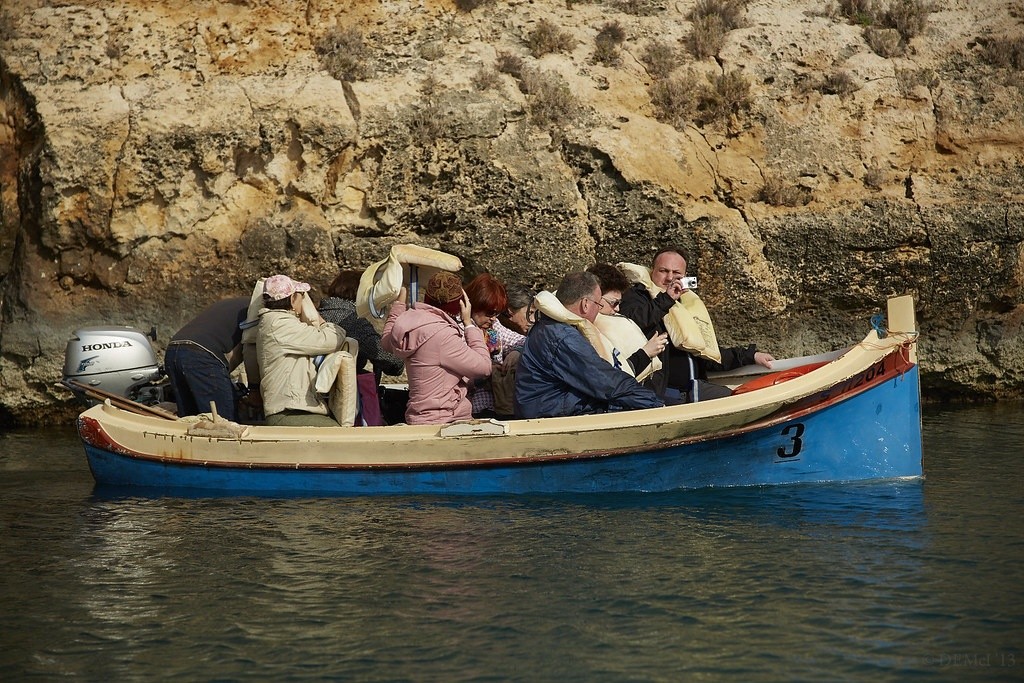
[171, 343, 204, 351]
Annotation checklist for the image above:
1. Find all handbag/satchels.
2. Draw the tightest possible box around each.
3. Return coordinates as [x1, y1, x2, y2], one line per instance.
[492, 357, 517, 415]
[328, 341, 356, 427]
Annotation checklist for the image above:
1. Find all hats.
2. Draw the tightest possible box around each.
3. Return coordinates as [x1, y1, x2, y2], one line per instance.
[262, 275, 311, 301]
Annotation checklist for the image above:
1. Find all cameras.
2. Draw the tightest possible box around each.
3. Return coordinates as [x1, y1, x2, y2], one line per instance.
[680, 276, 697, 290]
[663, 340, 669, 347]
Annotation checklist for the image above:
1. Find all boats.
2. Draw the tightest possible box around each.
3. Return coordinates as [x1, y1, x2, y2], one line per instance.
[56, 291, 931, 503]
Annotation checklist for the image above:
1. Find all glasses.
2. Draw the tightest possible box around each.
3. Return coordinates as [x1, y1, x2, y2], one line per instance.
[581, 297, 604, 309]
[485, 309, 501, 317]
[602, 296, 623, 307]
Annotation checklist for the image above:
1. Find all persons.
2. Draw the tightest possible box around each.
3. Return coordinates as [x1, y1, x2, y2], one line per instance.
[449, 273, 527, 419]
[255, 275, 346, 427]
[617, 247, 776, 406]
[491, 283, 538, 420]
[316, 271, 404, 376]
[164, 296, 251, 422]
[586, 263, 669, 400]
[513, 271, 667, 420]
[381, 271, 492, 425]
